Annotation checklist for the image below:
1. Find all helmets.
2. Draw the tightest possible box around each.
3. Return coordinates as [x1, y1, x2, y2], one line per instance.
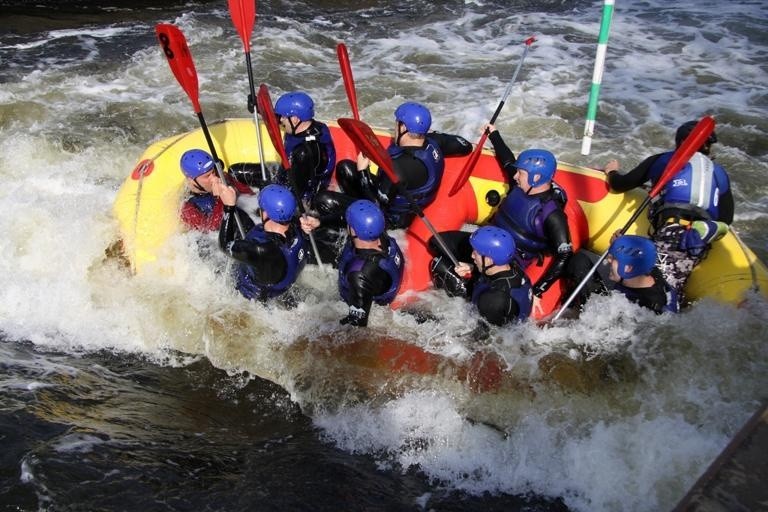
[257, 183, 298, 223]
[393, 102, 432, 136]
[273, 91, 315, 123]
[511, 147, 557, 189]
[468, 224, 517, 267]
[674, 117, 717, 156]
[346, 198, 386, 241]
[179, 148, 216, 180]
[606, 234, 658, 280]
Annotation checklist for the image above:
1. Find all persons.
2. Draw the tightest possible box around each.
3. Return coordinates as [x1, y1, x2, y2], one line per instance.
[216, 185, 307, 302]
[227, 91, 335, 210]
[315, 101, 472, 229]
[604, 121, 736, 299]
[180, 148, 254, 235]
[425, 123, 572, 320]
[400, 224, 535, 345]
[537, 228, 669, 329]
[298, 199, 407, 328]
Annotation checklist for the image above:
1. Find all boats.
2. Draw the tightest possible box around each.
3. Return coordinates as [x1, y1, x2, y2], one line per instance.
[114, 116, 768, 443]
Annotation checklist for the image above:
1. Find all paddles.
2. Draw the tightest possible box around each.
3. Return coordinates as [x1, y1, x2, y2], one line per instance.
[338, 118, 461, 270]
[336, 43, 382, 210]
[550, 115, 715, 326]
[155, 23, 245, 245]
[256, 85, 323, 270]
[228, 1, 266, 183]
[449, 38, 532, 196]
